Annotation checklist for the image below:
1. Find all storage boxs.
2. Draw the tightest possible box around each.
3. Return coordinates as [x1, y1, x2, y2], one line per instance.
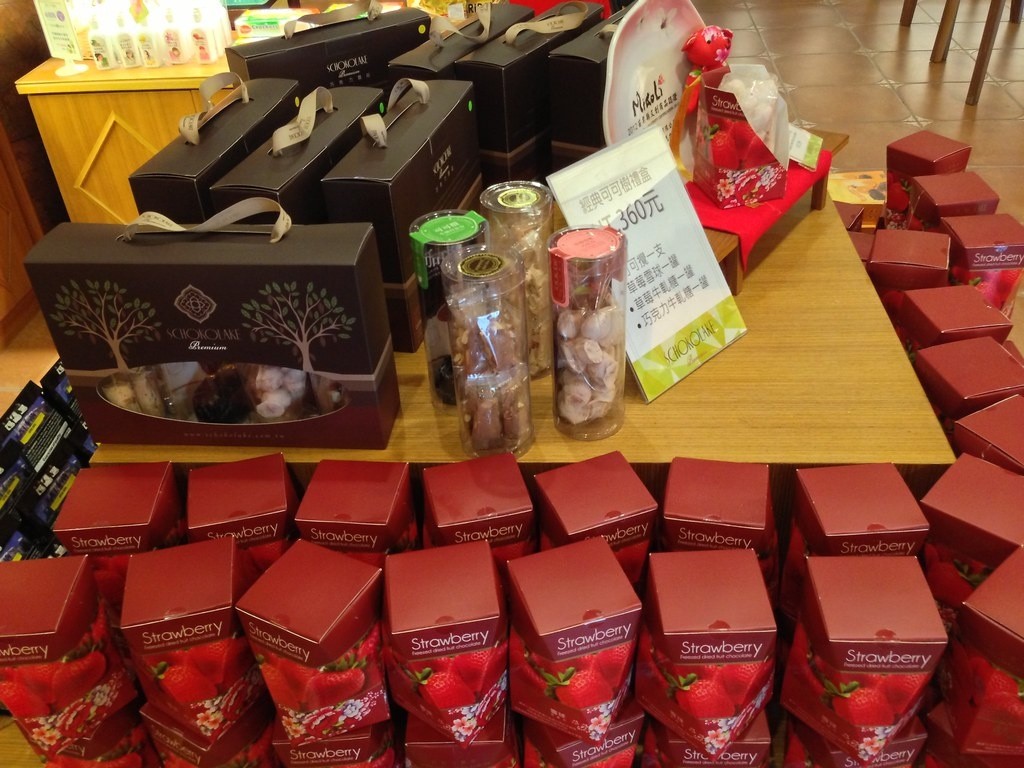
[120, 536, 267, 743]
[128, 78, 302, 223]
[386, 4, 535, 93]
[225, 7, 432, 106]
[33, 0, 320, 61]
[383, 129, 1024, 768]
[139, 701, 280, 768]
[186, 451, 298, 571]
[45, 726, 163, 768]
[209, 86, 384, 224]
[455, 1, 604, 190]
[51, 460, 186, 659]
[693, 67, 787, 210]
[293, 459, 419, 567]
[235, 539, 392, 749]
[0, 554, 138, 762]
[547, 0, 638, 173]
[22, 223, 400, 451]
[271, 713, 405, 768]
[319, 80, 486, 353]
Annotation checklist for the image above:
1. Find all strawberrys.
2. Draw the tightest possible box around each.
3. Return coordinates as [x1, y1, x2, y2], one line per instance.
[0, 118, 1024, 768]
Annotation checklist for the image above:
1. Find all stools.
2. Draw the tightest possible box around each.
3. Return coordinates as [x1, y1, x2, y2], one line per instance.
[703, 130, 850, 296]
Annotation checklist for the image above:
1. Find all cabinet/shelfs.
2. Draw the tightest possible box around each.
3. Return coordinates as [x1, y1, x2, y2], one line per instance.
[89, 186, 956, 564]
[14, 30, 239, 225]
